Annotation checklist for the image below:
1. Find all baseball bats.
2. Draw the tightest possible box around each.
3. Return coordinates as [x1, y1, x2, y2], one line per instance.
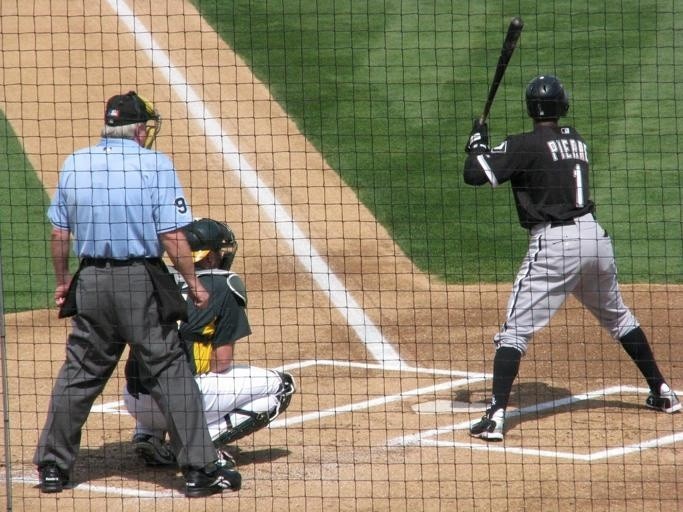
[479, 16, 524, 127]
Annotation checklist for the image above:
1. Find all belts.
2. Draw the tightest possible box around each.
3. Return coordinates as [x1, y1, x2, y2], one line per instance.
[85, 258, 143, 267]
[549, 212, 598, 228]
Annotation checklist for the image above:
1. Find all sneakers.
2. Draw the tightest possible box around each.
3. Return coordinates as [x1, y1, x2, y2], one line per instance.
[39, 465, 70, 491]
[471, 415, 504, 441]
[185, 467, 241, 496]
[132, 434, 174, 465]
[647, 389, 681, 414]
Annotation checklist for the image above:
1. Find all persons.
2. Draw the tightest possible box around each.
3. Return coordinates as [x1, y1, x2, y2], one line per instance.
[30, 91, 240, 498]
[122, 217, 295, 470]
[461, 76, 682, 442]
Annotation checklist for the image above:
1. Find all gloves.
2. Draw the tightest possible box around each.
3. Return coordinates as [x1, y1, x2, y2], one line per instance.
[468, 120, 489, 153]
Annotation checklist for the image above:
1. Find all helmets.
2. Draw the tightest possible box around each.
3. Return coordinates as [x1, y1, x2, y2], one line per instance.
[526, 75, 568, 120]
[105, 91, 160, 149]
[187, 218, 237, 270]
